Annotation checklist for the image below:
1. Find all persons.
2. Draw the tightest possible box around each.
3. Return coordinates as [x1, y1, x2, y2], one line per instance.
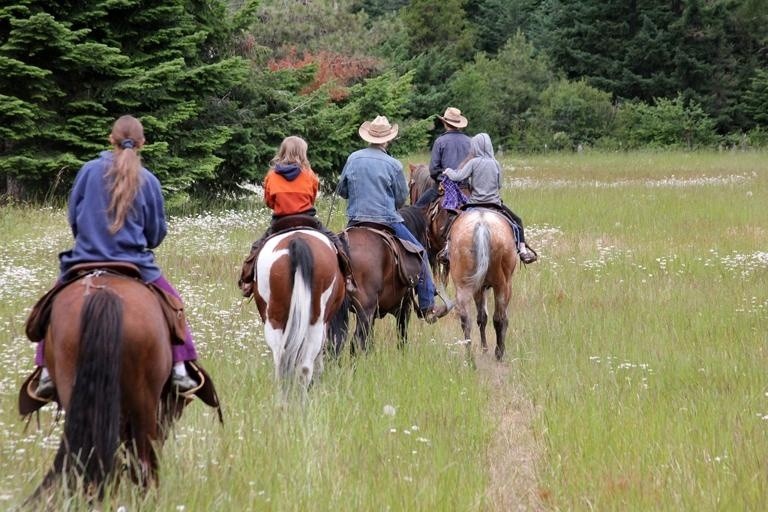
[243, 137, 356, 294]
[34, 114, 198, 393]
[438, 133, 533, 263]
[338, 116, 446, 323]
[416, 107, 471, 207]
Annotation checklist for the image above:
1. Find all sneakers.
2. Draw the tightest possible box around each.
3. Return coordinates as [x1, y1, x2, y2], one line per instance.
[347, 282, 354, 291]
[240, 281, 253, 297]
[520, 250, 536, 262]
[423, 306, 447, 323]
[169, 374, 197, 392]
[438, 248, 448, 261]
[35, 376, 54, 399]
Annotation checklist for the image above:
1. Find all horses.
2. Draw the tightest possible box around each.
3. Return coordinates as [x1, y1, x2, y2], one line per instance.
[252, 224, 346, 403]
[24, 272, 194, 506]
[408, 163, 471, 291]
[330, 202, 431, 379]
[447, 207, 518, 372]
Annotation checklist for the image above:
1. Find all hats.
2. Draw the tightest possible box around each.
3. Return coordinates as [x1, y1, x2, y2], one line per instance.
[359, 115, 400, 144]
[435, 106, 469, 129]
